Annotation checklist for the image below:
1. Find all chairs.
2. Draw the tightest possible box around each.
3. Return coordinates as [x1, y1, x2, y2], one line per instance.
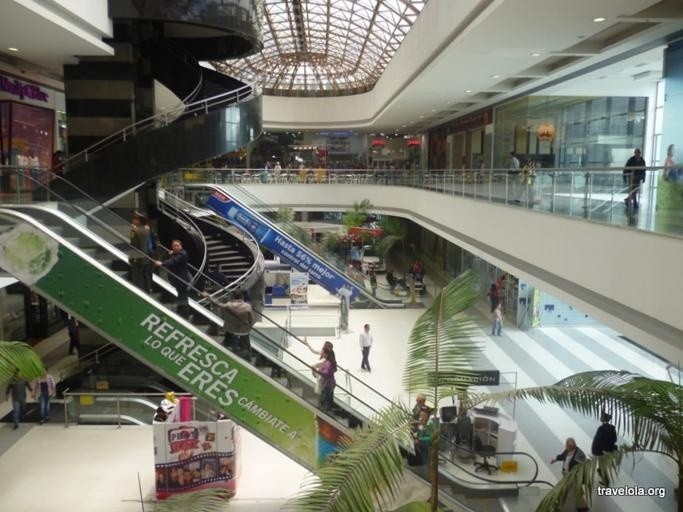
[452, 422, 473, 452]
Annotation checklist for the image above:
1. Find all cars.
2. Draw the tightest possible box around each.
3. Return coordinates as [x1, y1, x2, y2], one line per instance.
[360, 246, 386, 274]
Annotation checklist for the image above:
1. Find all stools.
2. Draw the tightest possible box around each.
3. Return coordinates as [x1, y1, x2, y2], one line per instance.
[214, 171, 456, 185]
[473, 445, 499, 475]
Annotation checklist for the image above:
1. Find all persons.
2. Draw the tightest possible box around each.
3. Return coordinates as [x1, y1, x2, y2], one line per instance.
[661, 144, 678, 181]
[491, 303, 503, 336]
[32, 366, 56, 425]
[623, 148, 646, 209]
[488, 283, 499, 313]
[129, 209, 256, 362]
[409, 393, 433, 468]
[312, 342, 337, 412]
[359, 324, 372, 372]
[359, 260, 427, 297]
[67, 316, 79, 356]
[15, 147, 41, 190]
[4, 367, 34, 430]
[550, 438, 588, 496]
[504, 151, 536, 208]
[591, 412, 617, 489]
